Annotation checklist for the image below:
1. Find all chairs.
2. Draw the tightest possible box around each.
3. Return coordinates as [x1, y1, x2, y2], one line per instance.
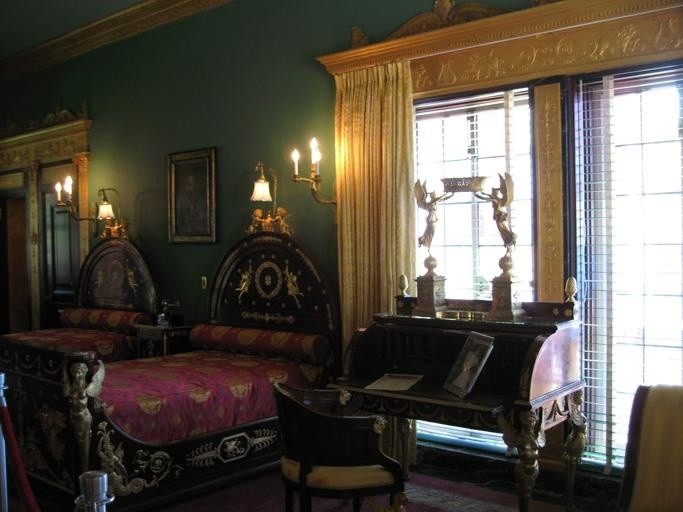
[271, 378, 411, 512]
[612, 382, 682, 512]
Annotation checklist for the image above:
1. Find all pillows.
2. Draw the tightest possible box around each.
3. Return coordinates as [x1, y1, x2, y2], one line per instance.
[56, 304, 149, 334]
[187, 321, 332, 368]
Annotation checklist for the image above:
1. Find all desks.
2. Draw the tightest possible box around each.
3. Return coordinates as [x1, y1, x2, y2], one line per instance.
[322, 294, 588, 512]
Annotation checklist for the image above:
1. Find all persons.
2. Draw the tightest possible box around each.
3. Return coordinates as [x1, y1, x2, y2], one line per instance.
[449, 339, 485, 390]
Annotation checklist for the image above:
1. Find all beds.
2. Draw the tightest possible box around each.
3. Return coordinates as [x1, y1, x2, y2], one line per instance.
[0, 236, 162, 368]
[0, 232, 340, 510]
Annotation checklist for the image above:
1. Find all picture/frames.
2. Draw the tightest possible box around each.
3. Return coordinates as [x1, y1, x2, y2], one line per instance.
[440, 329, 496, 401]
[362, 371, 426, 397]
[165, 145, 218, 246]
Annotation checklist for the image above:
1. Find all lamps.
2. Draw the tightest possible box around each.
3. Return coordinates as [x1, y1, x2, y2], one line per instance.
[52, 173, 98, 235]
[286, 135, 336, 209]
[237, 159, 293, 236]
[95, 186, 134, 242]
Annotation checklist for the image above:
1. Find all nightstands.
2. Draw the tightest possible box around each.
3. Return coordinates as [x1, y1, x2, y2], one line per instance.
[135, 323, 197, 360]
